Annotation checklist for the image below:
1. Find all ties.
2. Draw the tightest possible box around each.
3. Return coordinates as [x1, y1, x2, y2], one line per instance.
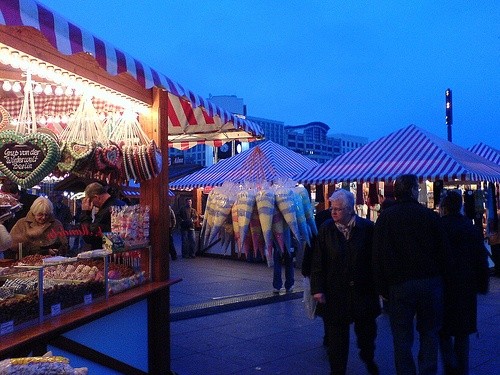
[344, 226, 349, 240]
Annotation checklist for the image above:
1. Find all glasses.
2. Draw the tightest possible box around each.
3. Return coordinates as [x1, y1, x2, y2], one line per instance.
[329, 207, 345, 212]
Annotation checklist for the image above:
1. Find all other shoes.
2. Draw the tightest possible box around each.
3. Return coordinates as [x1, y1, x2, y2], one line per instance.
[286, 287, 293, 292]
[365, 360, 380, 375]
[173, 257, 177, 261]
[184, 254, 196, 258]
[273, 288, 279, 293]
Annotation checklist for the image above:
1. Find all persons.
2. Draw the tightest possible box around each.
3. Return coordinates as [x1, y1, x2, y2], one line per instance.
[179, 196, 198, 259]
[272, 226, 295, 292]
[301, 188, 381, 375]
[0, 171, 128, 257]
[168, 204, 177, 261]
[365, 174, 500, 375]
